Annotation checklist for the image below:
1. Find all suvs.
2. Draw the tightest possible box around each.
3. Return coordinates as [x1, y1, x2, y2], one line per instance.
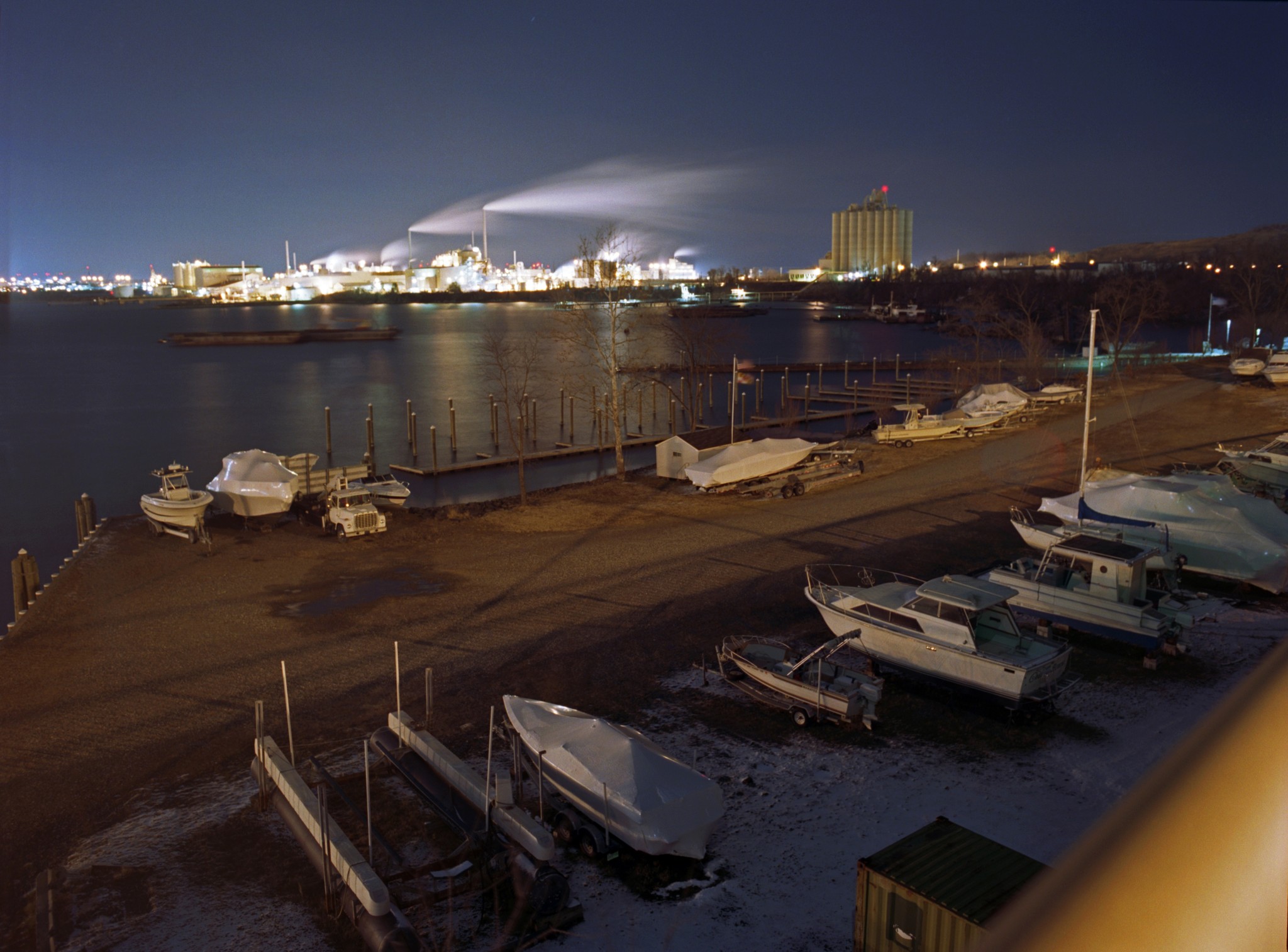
[321, 487, 388, 538]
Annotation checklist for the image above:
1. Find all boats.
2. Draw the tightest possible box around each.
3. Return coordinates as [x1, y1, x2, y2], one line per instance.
[801, 559, 1073, 702]
[501, 693, 726, 861]
[158, 327, 409, 346]
[718, 627, 891, 732]
[1036, 304, 1288, 592]
[1260, 350, 1288, 384]
[1214, 432, 1288, 493]
[684, 437, 819, 486]
[205, 447, 300, 518]
[872, 382, 1083, 449]
[348, 472, 412, 509]
[976, 528, 1211, 650]
[139, 460, 215, 529]
[1228, 357, 1266, 377]
